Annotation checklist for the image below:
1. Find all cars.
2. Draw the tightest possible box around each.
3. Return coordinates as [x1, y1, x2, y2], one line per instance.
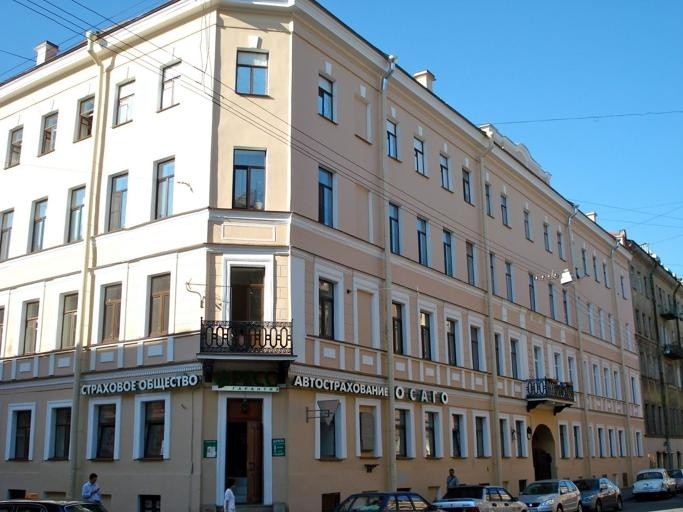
[0, 498, 109, 512]
[631, 468, 683, 499]
[336, 477, 623, 512]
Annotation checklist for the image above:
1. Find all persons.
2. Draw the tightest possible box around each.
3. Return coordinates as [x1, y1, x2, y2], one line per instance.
[223, 479, 236, 512]
[447, 469, 459, 487]
[81, 473, 101, 503]
